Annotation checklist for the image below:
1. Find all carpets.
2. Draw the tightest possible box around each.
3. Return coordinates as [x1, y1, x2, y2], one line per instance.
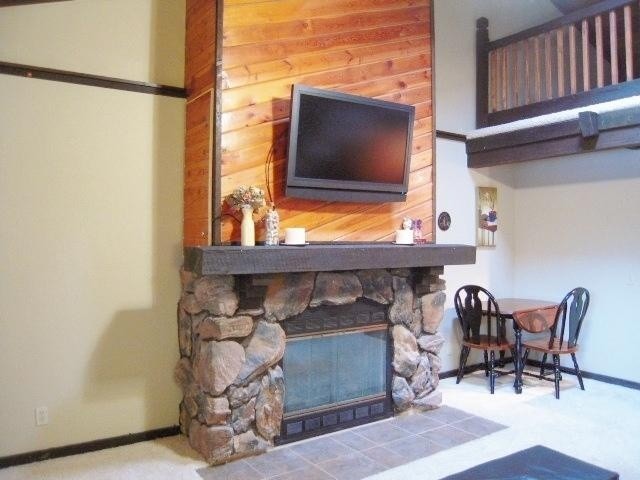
[440, 445, 620, 480]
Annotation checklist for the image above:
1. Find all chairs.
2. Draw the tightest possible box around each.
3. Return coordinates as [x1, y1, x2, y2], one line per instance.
[453, 284, 521, 394]
[514, 287, 590, 398]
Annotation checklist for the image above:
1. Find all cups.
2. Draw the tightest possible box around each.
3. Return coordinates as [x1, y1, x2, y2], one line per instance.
[285, 227, 306, 243]
[396, 230, 414, 244]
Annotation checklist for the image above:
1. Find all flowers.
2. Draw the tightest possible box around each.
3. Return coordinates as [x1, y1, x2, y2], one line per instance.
[225, 184, 266, 214]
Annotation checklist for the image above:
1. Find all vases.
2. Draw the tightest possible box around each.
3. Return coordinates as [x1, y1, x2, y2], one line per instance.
[240, 207, 256, 246]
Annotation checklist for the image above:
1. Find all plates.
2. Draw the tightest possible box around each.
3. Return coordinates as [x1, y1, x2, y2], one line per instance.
[391, 241, 417, 246]
[280, 242, 310, 247]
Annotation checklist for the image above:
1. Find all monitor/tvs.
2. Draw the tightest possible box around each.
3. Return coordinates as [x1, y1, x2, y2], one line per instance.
[284, 82, 416, 203]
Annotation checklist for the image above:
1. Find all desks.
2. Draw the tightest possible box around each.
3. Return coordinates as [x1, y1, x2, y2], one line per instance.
[462, 298, 561, 393]
